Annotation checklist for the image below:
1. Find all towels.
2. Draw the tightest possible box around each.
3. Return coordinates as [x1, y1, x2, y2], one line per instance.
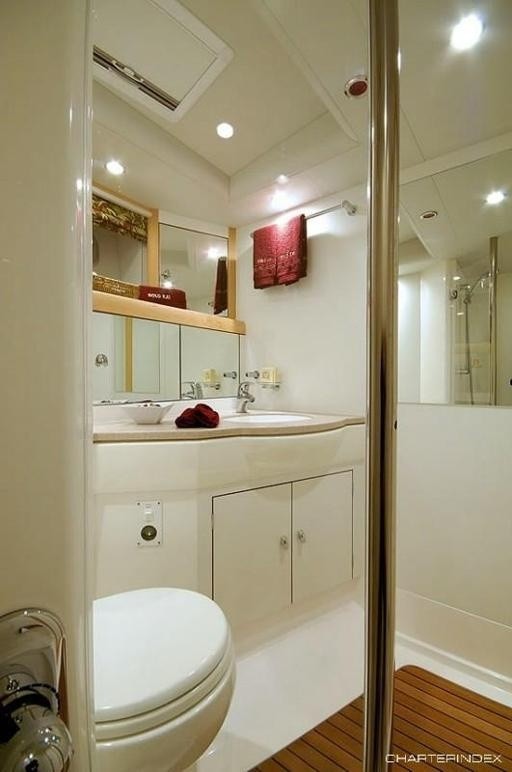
[251, 213, 308, 290]
[175, 402, 219, 428]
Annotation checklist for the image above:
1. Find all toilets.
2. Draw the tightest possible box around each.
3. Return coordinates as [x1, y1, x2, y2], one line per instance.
[91, 587, 239, 772]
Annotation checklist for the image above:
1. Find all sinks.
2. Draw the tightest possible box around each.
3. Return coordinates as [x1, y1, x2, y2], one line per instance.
[222, 413, 313, 423]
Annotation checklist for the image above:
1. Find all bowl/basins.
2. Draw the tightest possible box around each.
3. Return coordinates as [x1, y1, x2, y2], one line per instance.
[119, 402, 175, 425]
[92, 399, 130, 406]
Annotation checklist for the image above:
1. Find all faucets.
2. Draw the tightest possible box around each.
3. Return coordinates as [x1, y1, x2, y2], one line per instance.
[181, 381, 203, 400]
[235, 382, 254, 413]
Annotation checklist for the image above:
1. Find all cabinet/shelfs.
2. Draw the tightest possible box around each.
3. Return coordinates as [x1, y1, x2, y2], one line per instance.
[211, 470, 353, 656]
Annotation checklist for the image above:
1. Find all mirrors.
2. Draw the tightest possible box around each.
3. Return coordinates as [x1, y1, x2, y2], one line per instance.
[398, 149, 512, 407]
[91, 184, 247, 405]
